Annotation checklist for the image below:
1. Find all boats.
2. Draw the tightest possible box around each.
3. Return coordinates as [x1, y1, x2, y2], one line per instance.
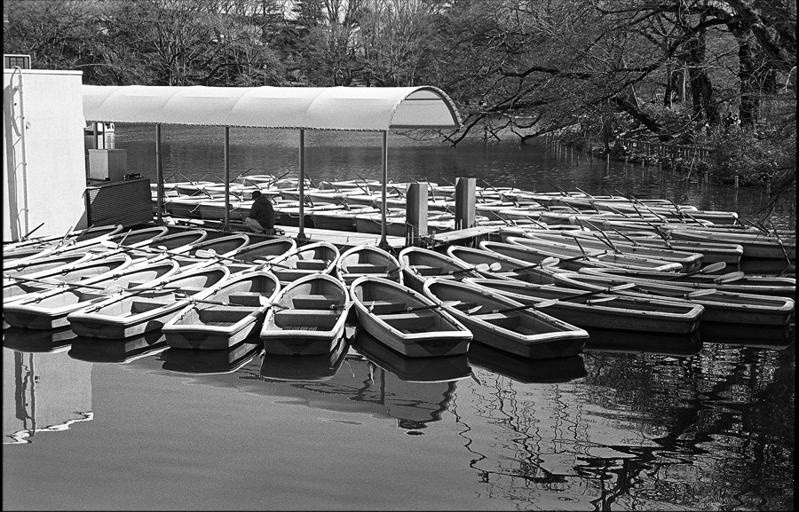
[474, 180, 738, 219]
[424, 222, 795, 360]
[147, 170, 460, 233]
[3, 217, 473, 362]
[3, 320, 795, 385]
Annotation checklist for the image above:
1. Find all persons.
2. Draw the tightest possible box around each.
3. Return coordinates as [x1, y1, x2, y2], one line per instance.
[245, 191, 275, 233]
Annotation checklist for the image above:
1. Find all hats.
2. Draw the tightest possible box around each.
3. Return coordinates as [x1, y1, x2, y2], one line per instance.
[250, 191, 261, 199]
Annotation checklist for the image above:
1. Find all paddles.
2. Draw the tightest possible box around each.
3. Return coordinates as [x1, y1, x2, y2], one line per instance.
[388, 300, 461, 314]
[433, 263, 488, 274]
[471, 299, 557, 312]
[40, 278, 106, 290]
[491, 262, 502, 270]
[508, 257, 561, 270]
[686, 272, 744, 284]
[559, 284, 636, 300]
[648, 289, 719, 299]
[196, 249, 249, 263]
[667, 262, 726, 279]
[101, 240, 162, 254]
[123, 287, 210, 291]
